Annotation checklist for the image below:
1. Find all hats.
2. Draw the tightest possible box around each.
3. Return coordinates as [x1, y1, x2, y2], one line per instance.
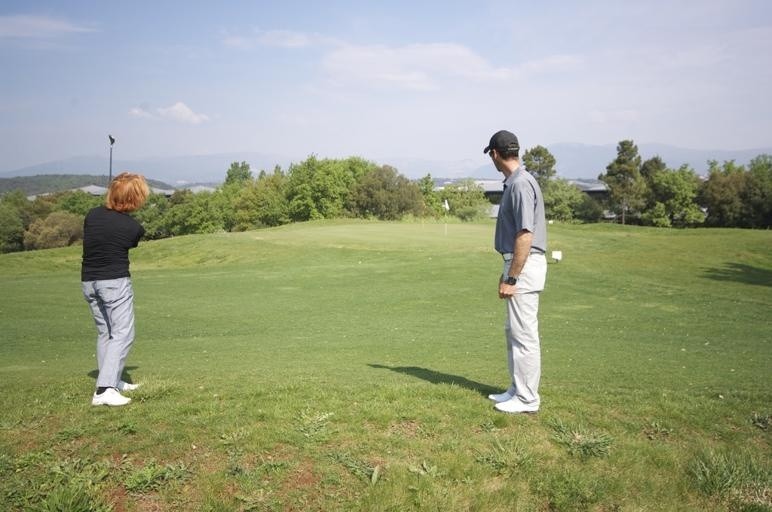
[483, 130, 519, 154]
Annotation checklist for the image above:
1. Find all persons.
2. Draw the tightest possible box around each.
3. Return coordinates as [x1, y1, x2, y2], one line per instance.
[82, 172, 152, 406]
[484, 129, 549, 415]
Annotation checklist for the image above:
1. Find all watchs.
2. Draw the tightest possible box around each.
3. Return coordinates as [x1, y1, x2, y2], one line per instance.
[503, 276, 516, 285]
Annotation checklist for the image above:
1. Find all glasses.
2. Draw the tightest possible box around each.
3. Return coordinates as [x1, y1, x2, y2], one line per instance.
[489, 151, 494, 156]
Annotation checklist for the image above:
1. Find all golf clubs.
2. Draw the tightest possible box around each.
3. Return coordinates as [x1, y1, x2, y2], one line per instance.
[108, 134, 115, 184]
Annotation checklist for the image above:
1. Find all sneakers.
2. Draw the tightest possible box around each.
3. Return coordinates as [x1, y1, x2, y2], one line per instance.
[488, 392, 540, 413]
[92, 380, 139, 406]
